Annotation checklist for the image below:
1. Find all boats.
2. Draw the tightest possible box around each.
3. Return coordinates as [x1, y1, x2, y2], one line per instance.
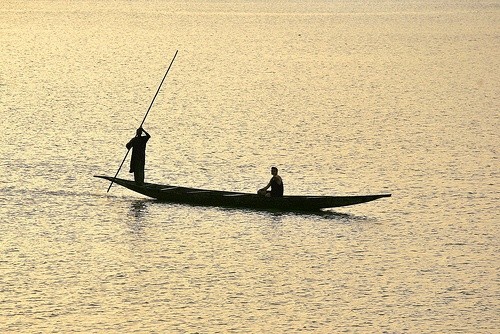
[94, 176, 392, 210]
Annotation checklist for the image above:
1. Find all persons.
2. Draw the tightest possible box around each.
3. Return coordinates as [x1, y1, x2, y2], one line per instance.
[256, 167, 284, 198]
[125, 127, 151, 184]
